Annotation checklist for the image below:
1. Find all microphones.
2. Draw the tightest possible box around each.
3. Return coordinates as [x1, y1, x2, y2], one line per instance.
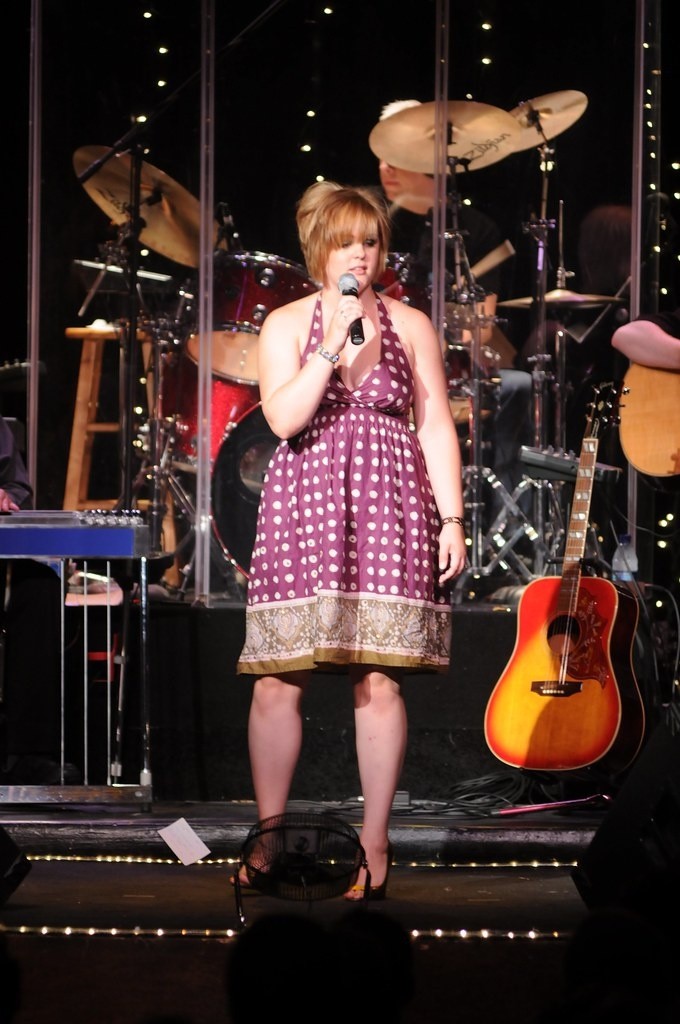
[339, 273, 366, 346]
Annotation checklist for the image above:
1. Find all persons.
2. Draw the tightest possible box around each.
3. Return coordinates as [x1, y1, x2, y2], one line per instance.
[230, 182, 467, 900]
[379, 158, 533, 482]
[611, 313, 680, 370]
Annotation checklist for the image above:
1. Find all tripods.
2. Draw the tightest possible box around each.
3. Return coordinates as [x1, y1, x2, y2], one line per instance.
[112, 254, 254, 606]
[440, 137, 607, 604]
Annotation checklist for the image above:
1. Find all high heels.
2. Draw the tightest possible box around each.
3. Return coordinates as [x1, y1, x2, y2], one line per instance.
[229, 829, 284, 887]
[343, 840, 392, 902]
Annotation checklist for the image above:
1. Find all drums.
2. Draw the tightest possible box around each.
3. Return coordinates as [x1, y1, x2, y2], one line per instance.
[133, 348, 262, 476]
[371, 252, 432, 319]
[443, 344, 501, 425]
[180, 250, 323, 386]
[210, 401, 282, 580]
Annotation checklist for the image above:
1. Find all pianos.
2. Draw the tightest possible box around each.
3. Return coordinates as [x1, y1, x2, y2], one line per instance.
[0, 510, 154, 812]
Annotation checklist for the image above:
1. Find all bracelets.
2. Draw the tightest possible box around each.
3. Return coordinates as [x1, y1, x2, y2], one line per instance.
[316, 343, 339, 363]
[441, 517, 465, 529]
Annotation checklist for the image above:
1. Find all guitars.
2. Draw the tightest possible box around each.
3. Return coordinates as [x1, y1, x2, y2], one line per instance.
[483, 381, 645, 782]
[619, 363, 680, 478]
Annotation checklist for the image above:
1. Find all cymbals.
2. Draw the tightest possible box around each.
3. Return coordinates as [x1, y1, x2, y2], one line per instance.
[369, 100, 521, 175]
[72, 145, 228, 270]
[495, 289, 626, 311]
[508, 90, 588, 152]
